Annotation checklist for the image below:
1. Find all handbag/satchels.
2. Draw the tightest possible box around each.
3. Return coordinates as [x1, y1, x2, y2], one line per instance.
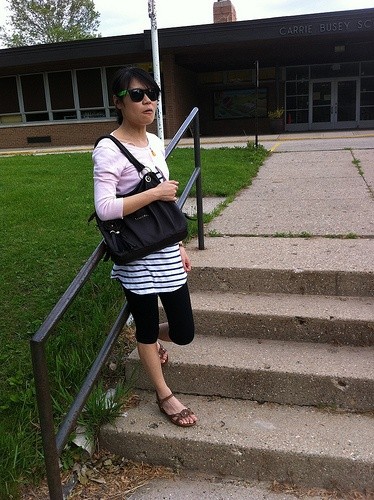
[87, 136, 189, 264]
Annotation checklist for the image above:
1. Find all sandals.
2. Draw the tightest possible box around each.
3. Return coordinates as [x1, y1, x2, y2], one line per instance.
[156, 389, 197, 426]
[156, 342, 168, 366]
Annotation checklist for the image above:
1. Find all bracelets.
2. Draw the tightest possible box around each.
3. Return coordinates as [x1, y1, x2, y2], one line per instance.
[179, 244, 184, 247]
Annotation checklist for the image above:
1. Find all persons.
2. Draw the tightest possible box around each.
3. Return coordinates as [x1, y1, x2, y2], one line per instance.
[92, 65, 199, 427]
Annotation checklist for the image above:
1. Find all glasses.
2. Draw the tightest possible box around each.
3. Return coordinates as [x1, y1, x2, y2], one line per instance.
[116, 88, 158, 102]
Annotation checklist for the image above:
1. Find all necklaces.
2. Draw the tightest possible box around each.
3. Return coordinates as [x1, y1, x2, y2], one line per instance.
[122, 128, 156, 157]
